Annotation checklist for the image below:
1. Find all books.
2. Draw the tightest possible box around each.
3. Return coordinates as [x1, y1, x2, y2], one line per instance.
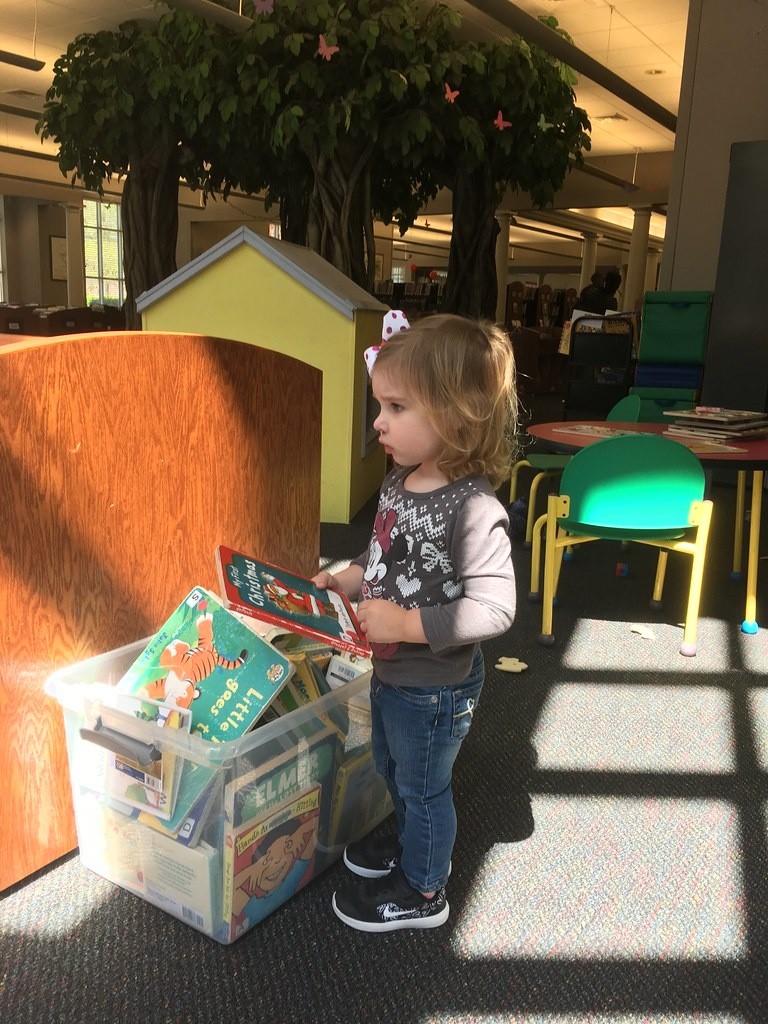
[662, 403, 768, 443]
[110, 544, 387, 942]
[556, 306, 641, 361]
[380, 272, 574, 330]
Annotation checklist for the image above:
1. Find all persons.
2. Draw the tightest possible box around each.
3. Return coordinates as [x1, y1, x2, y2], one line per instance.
[575, 273, 622, 313]
[581, 273, 603, 298]
[310, 314, 520, 933]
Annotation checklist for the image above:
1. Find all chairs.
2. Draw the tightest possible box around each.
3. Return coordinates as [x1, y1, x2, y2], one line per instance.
[507, 395, 641, 548]
[527, 433, 715, 657]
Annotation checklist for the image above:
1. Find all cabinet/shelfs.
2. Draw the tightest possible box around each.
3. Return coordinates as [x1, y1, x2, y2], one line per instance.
[368, 278, 579, 394]
[1, 301, 129, 338]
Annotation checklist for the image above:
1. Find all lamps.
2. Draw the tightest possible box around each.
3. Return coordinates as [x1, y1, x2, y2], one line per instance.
[0, 1, 46, 71]
[566, 146, 642, 193]
[461, 1, 677, 133]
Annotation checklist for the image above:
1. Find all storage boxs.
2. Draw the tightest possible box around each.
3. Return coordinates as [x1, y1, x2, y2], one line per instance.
[629, 290, 714, 423]
[43, 612, 399, 945]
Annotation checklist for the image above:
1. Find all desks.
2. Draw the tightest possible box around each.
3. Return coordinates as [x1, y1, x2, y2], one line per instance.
[523, 422, 768, 630]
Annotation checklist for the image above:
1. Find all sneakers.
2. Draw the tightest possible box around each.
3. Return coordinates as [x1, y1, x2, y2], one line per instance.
[344, 834, 453, 878]
[331, 864, 450, 932]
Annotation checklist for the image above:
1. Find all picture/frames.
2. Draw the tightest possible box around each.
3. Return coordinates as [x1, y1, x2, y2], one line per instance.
[50, 235, 68, 281]
[364, 252, 384, 281]
